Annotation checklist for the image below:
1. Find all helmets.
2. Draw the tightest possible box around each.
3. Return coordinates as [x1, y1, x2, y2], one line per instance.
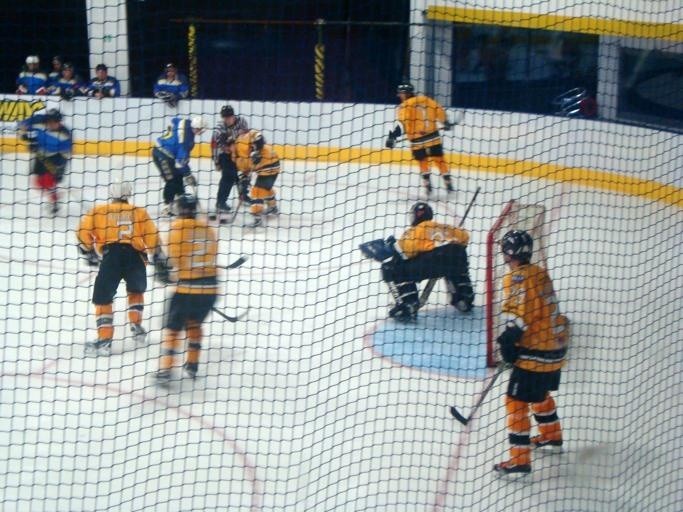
[168, 193, 197, 218]
[395, 83, 414, 94]
[217, 132, 235, 147]
[108, 177, 133, 204]
[164, 62, 176, 72]
[493, 230, 533, 262]
[191, 115, 209, 136]
[220, 105, 234, 118]
[42, 109, 63, 122]
[408, 203, 432, 227]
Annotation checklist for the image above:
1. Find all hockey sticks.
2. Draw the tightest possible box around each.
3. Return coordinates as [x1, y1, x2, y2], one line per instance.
[449, 361, 507, 426]
[395, 109, 464, 145]
[88, 255, 249, 271]
[191, 184, 217, 222]
[210, 306, 249, 324]
[221, 163, 257, 225]
[420, 186, 481, 306]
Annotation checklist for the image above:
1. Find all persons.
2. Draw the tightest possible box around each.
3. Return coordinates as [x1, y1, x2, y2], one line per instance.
[22, 107, 74, 213]
[15, 53, 199, 103]
[77, 182, 163, 350]
[491, 231, 569, 475]
[211, 105, 251, 212]
[152, 192, 218, 385]
[577, 90, 597, 121]
[152, 113, 207, 216]
[222, 131, 281, 232]
[386, 81, 453, 195]
[381, 204, 475, 327]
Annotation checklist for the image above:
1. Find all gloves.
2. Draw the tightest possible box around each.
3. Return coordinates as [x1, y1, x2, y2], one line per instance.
[443, 121, 450, 131]
[359, 236, 395, 262]
[77, 244, 101, 266]
[496, 320, 525, 363]
[155, 251, 171, 270]
[155, 91, 178, 109]
[42, 154, 64, 171]
[184, 173, 198, 187]
[249, 149, 263, 163]
[22, 134, 36, 151]
[385, 131, 396, 147]
[153, 273, 173, 285]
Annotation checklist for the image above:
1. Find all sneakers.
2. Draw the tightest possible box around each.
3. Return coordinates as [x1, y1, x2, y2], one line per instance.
[215, 199, 232, 211]
[91, 339, 110, 351]
[239, 190, 252, 202]
[529, 430, 562, 447]
[494, 457, 531, 473]
[150, 370, 170, 378]
[249, 217, 262, 227]
[161, 205, 175, 216]
[458, 300, 475, 312]
[131, 322, 145, 334]
[262, 206, 279, 215]
[390, 302, 418, 316]
[182, 362, 197, 379]
